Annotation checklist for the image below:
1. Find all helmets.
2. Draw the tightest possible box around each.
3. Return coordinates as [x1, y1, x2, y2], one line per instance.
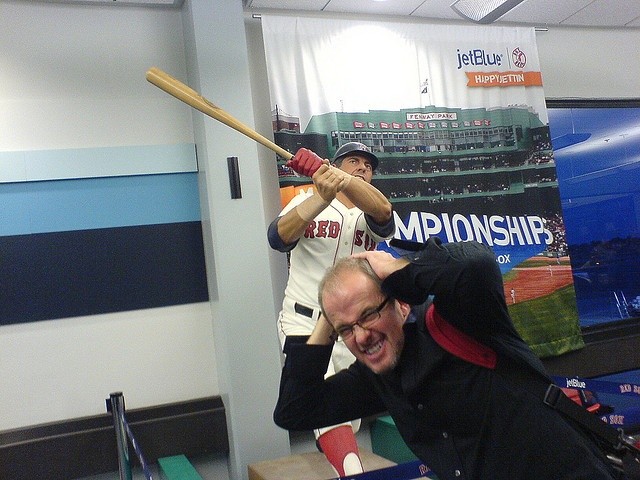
[333, 140, 380, 172]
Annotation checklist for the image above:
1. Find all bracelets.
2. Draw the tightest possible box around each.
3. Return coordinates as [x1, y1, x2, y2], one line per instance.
[328, 166, 353, 193]
[296, 193, 331, 225]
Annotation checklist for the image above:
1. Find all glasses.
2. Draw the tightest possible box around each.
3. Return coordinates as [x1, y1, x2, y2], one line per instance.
[328, 296, 393, 344]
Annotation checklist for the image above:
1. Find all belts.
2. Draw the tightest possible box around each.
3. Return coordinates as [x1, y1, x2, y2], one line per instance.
[295, 302, 322, 320]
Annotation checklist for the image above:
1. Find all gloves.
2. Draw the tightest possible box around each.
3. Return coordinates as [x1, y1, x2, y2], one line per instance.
[284, 146, 324, 178]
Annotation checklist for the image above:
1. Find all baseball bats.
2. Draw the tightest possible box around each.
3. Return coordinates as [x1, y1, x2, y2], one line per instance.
[145, 66, 345, 192]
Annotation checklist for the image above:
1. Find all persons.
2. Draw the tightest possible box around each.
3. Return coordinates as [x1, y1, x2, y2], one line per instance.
[274, 236, 639, 480]
[267, 142, 396, 479]
